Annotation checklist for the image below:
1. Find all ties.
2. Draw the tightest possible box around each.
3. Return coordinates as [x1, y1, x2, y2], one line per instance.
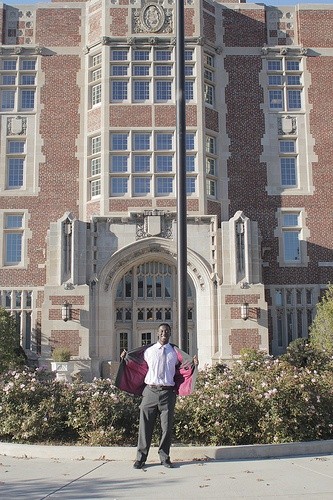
[158, 347, 166, 379]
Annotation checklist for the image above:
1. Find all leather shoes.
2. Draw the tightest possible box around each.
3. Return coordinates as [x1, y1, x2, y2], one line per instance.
[161, 462, 172, 468]
[134, 461, 145, 469]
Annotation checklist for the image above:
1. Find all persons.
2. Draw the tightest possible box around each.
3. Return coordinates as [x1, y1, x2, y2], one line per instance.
[117, 323, 199, 468]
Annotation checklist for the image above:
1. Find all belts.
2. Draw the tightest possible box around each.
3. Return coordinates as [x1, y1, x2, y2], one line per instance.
[152, 385, 174, 390]
